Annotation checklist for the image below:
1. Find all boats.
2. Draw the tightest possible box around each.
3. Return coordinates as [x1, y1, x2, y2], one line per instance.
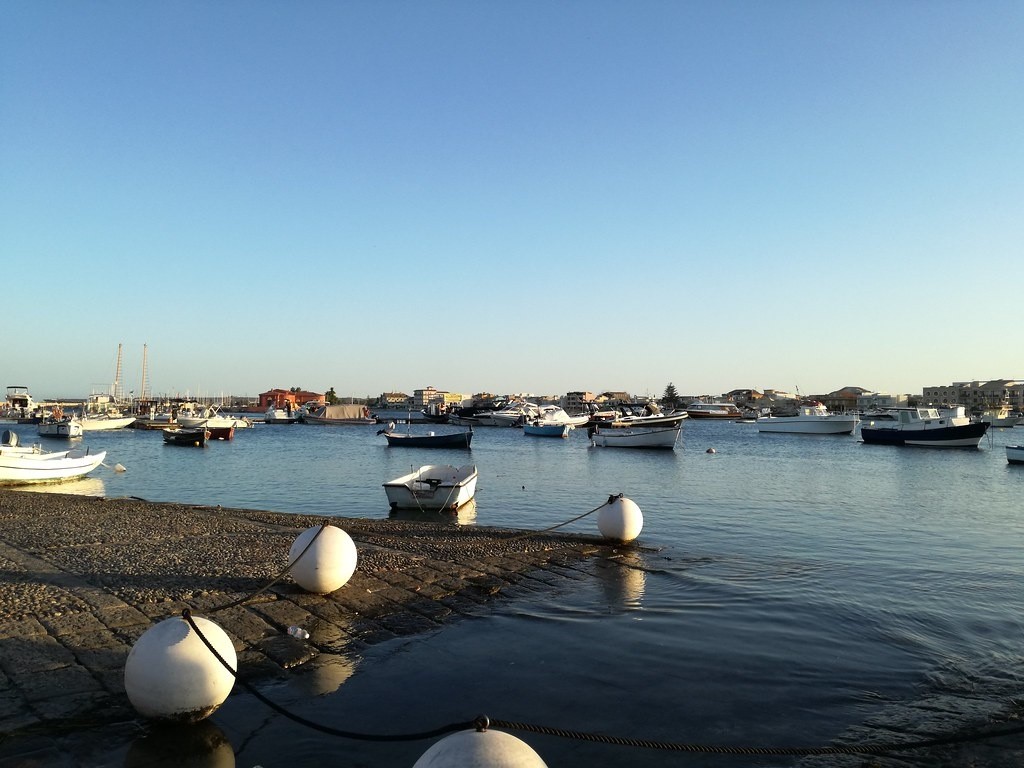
[683, 404, 744, 419]
[0, 376, 330, 447]
[747, 400, 1024, 448]
[1006, 444, 1024, 465]
[420, 397, 689, 449]
[383, 463, 478, 510]
[385, 423, 474, 448]
[0, 451, 107, 485]
[0, 444, 71, 461]
[305, 405, 376, 425]
